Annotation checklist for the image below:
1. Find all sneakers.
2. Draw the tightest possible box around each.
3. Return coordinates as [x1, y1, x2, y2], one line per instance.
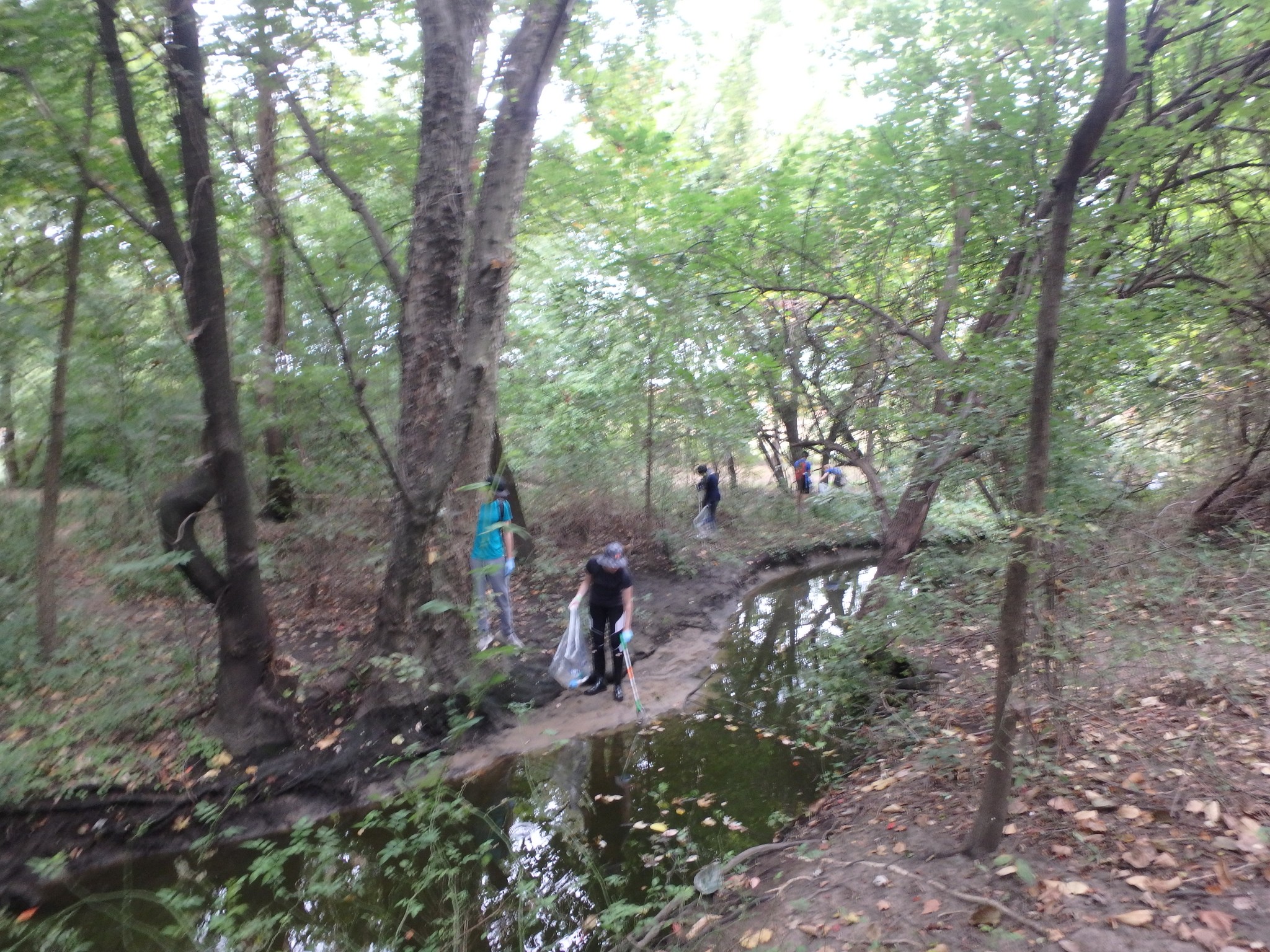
[495, 631, 526, 650]
[475, 633, 495, 652]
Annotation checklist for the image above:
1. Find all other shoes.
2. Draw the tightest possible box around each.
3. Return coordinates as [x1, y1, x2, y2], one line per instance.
[614, 683, 624, 702]
[584, 677, 607, 696]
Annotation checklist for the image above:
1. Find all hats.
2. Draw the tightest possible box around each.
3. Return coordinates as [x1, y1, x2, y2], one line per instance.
[486, 474, 511, 497]
[597, 542, 627, 569]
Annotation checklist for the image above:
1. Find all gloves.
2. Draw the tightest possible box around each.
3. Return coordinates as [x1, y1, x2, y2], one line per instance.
[504, 557, 515, 577]
[622, 630, 633, 643]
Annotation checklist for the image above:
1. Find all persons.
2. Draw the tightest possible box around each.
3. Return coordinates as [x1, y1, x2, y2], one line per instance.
[817, 463, 845, 489]
[793, 451, 811, 507]
[566, 542, 634, 702]
[468, 475, 526, 652]
[696, 465, 721, 525]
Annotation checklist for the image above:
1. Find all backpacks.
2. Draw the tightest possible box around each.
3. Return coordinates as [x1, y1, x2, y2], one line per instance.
[796, 462, 807, 482]
